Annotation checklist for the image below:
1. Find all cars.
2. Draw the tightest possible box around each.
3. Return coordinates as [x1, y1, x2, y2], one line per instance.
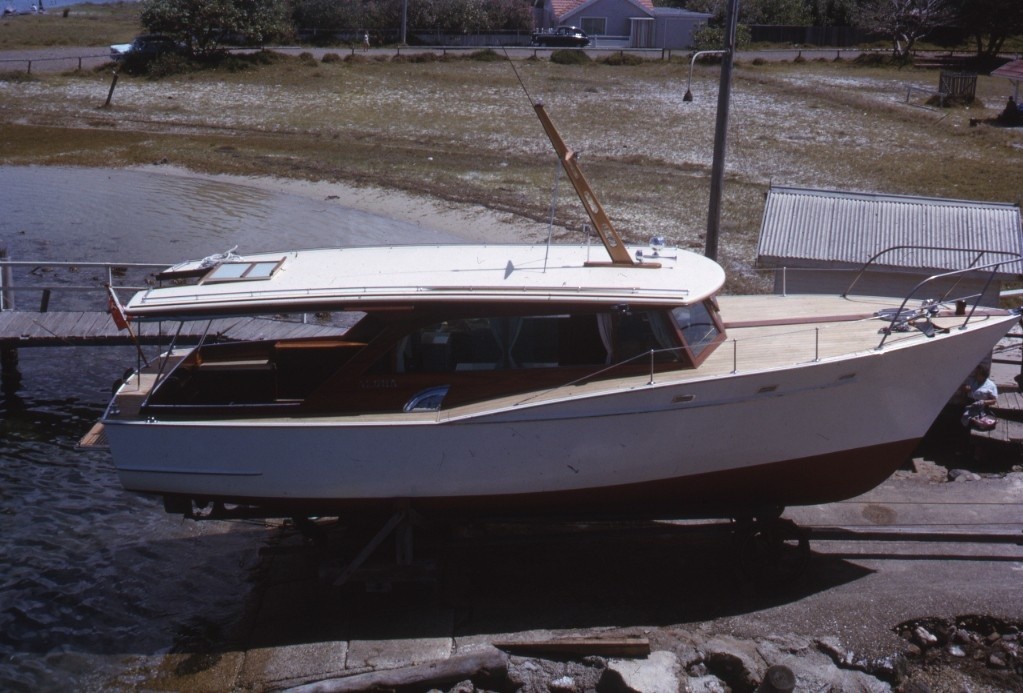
[532, 27, 590, 47]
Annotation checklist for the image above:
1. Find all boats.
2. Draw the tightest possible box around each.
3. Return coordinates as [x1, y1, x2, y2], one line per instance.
[72, 5, 1023, 518]
